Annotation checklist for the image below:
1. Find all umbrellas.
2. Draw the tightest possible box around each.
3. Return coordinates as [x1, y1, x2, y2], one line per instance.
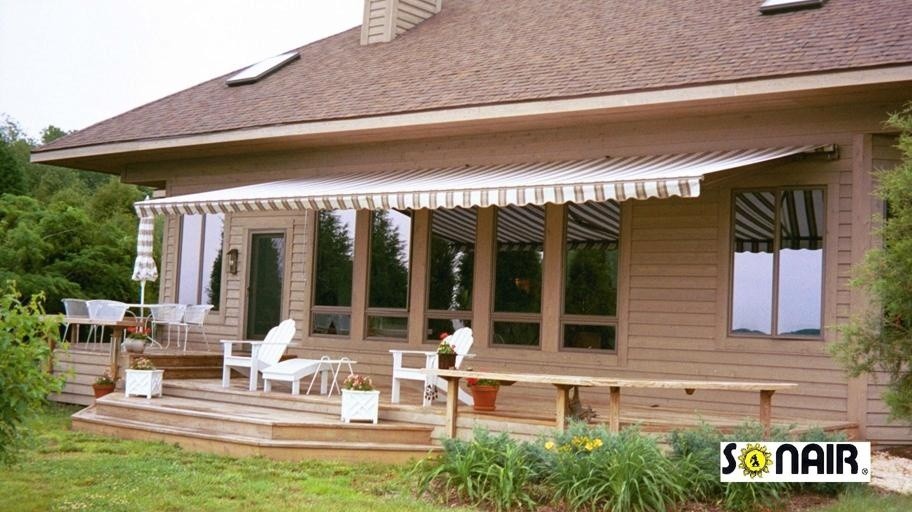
[129, 194, 160, 321]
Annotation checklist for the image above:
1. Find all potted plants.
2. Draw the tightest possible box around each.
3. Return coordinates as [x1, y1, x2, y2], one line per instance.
[93, 377, 116, 399]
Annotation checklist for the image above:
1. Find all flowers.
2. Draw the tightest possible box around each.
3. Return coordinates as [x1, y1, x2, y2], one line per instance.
[129, 354, 157, 370]
[466, 376, 502, 391]
[344, 373, 374, 391]
[438, 341, 456, 353]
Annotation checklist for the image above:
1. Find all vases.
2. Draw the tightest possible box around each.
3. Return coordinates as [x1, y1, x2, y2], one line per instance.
[439, 353, 457, 369]
[471, 386, 498, 411]
[340, 388, 381, 424]
[125, 369, 165, 399]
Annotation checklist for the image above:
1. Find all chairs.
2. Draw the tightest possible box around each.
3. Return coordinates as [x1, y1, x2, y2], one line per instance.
[220, 317, 296, 390]
[388, 326, 475, 408]
[60, 297, 216, 353]
